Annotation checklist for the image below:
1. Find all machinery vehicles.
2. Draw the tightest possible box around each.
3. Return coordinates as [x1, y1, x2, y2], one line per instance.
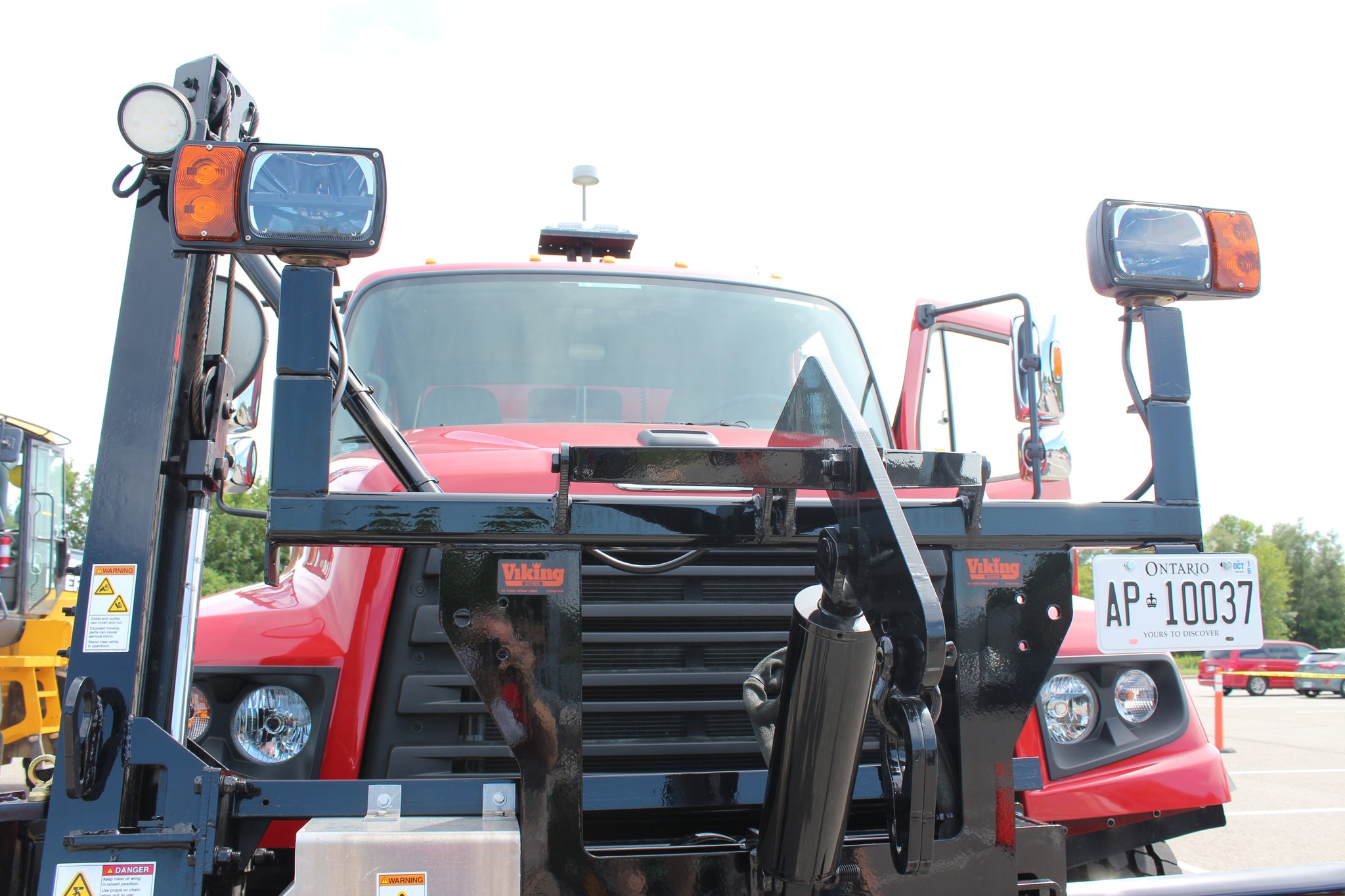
[1, 409, 95, 837]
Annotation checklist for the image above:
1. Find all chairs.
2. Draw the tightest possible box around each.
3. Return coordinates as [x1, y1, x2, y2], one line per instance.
[417, 385, 502, 428]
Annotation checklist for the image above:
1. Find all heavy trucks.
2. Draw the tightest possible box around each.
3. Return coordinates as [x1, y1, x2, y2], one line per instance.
[28, 54, 1344, 896]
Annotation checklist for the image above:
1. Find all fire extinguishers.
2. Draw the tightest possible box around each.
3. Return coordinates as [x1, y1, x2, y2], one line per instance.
[0, 528, 19, 571]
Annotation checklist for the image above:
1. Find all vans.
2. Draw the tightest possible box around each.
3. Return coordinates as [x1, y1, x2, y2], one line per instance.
[1198, 639, 1318, 697]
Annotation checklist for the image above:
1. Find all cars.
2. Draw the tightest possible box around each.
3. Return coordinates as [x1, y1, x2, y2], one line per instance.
[1292, 648, 1345, 698]
